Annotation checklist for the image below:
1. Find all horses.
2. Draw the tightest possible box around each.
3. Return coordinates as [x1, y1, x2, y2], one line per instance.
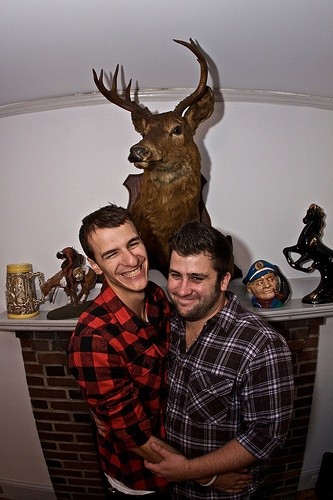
[282, 204, 333, 300]
[40, 265, 98, 307]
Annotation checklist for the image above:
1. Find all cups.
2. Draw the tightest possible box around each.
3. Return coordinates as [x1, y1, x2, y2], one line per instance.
[5, 264, 45, 319]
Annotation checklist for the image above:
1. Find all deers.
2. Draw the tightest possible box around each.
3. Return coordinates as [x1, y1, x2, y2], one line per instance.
[92, 37, 215, 281]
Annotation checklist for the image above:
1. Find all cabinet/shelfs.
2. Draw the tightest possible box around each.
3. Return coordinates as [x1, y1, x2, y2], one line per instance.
[0, 296, 332, 500]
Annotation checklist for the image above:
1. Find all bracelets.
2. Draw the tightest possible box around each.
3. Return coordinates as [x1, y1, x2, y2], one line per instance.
[198, 473, 218, 488]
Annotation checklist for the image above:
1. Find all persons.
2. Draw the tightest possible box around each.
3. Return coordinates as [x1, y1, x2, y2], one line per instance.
[142, 220, 296, 500]
[242, 260, 292, 308]
[67, 201, 255, 499]
